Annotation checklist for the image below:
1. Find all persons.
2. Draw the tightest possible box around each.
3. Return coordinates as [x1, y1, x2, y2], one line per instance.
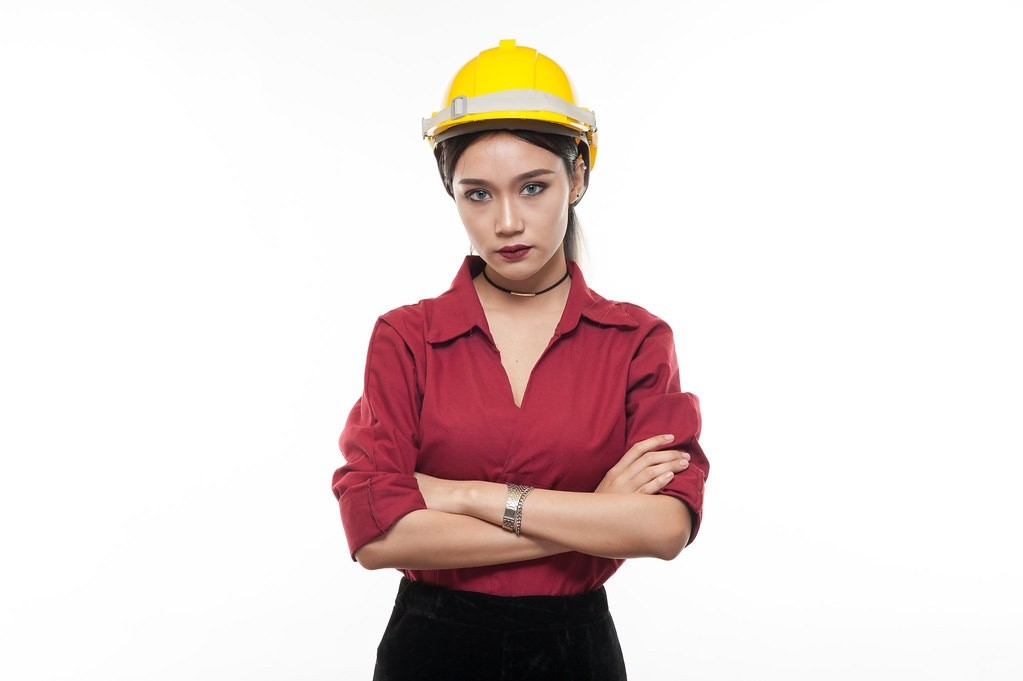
[332, 40, 708, 681]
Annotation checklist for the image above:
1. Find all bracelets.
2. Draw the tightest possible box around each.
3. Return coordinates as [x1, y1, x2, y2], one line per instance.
[502, 483, 535, 537]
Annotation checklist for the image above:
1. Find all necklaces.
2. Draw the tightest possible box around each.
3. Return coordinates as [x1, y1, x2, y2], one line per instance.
[483, 266, 569, 296]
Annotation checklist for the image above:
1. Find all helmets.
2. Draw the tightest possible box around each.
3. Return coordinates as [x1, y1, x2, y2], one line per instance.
[422, 40, 597, 171]
